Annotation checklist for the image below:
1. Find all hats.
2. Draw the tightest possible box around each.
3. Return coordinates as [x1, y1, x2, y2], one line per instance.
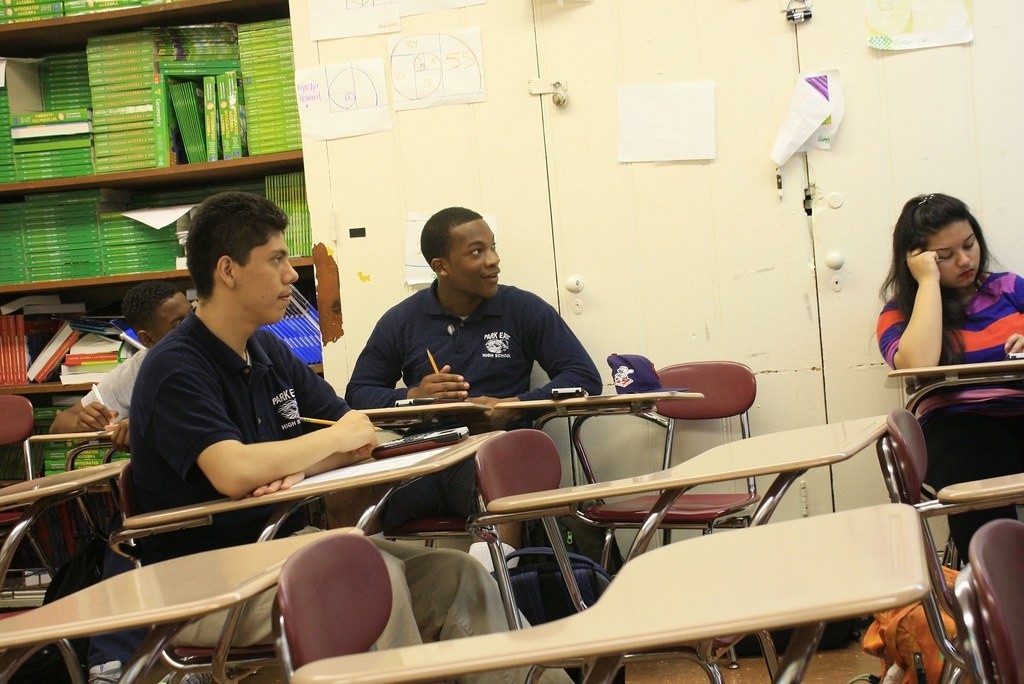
[607, 354, 690, 395]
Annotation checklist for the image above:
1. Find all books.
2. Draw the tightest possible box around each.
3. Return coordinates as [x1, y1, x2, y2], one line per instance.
[0, 0, 325, 476]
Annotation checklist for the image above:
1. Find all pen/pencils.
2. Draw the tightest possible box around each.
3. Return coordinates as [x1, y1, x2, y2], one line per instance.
[92, 383, 105, 405]
[937, 259, 944, 263]
[298, 416, 384, 432]
[426, 348, 439, 373]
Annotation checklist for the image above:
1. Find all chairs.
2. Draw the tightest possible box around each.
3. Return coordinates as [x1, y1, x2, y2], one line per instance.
[116, 357, 1024, 684]
[0, 395, 75, 557]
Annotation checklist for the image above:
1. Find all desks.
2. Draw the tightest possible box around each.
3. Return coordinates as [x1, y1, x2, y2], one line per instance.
[935, 471, 1024, 511]
[885, 359, 1024, 394]
[356, 389, 707, 506]
[475, 414, 889, 677]
[115, 430, 506, 684]
[0, 527, 363, 684]
[289, 499, 932, 684]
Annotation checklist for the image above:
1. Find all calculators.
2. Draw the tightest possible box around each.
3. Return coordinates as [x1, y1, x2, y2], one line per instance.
[549, 387, 589, 398]
[395, 397, 464, 407]
[1003, 352, 1024, 360]
[371, 426, 470, 460]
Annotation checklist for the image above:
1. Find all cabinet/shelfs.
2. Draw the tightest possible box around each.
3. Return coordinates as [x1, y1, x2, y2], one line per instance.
[0, 0, 322, 396]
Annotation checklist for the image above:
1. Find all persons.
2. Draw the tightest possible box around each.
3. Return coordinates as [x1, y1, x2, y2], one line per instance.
[343, 208, 602, 627]
[49, 281, 197, 684]
[877, 193, 1023, 566]
[127, 192, 577, 684]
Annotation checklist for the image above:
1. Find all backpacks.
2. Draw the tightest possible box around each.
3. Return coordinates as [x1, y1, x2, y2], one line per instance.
[521, 499, 624, 579]
[5, 533, 108, 684]
[862, 565, 971, 684]
[490, 547, 625, 684]
